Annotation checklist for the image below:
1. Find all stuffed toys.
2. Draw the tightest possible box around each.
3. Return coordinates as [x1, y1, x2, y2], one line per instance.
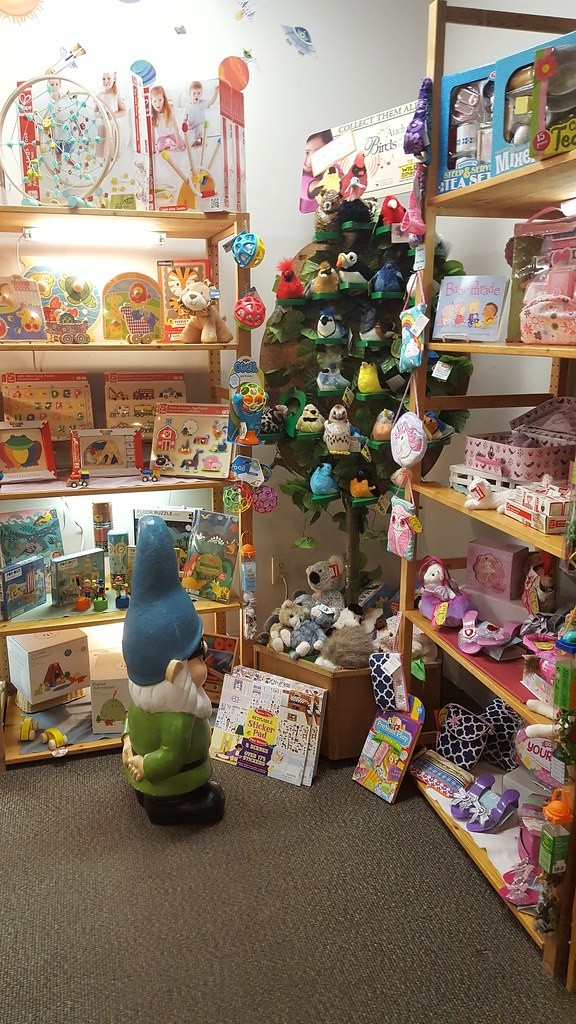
[177, 279, 233, 344]
[254, 562, 478, 671]
[260, 177, 453, 498]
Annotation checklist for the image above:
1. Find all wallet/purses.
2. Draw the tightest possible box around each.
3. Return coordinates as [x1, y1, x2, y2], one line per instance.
[406, 748, 474, 798]
[519, 292, 576, 345]
[368, 611, 411, 713]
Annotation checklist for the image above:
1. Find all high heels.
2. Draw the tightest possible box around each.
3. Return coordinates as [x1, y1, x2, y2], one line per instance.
[449, 774, 496, 820]
[476, 619, 524, 646]
[457, 609, 482, 656]
[466, 788, 521, 832]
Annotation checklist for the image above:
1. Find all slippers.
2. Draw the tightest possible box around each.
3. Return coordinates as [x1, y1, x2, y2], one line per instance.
[502, 857, 536, 888]
[499, 879, 541, 906]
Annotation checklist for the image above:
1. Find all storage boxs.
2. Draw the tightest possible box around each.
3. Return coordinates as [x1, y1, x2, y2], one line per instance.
[201, 631, 245, 703]
[438, 31, 575, 195]
[503, 765, 571, 878]
[0, 555, 46, 622]
[133, 505, 205, 566]
[17, 67, 248, 214]
[460, 540, 576, 712]
[5, 628, 90, 705]
[447, 394, 575, 536]
[51, 547, 106, 608]
[92, 651, 130, 735]
[0, 369, 236, 484]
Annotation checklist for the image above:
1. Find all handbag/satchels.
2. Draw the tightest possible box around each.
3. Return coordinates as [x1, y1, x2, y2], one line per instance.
[399, 272, 428, 374]
[435, 702, 493, 772]
[479, 695, 529, 772]
[390, 373, 427, 468]
[386, 474, 423, 561]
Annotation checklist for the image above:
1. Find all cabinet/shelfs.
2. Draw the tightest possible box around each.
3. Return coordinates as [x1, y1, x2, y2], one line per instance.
[0, 206, 252, 770]
[381, 0, 575, 980]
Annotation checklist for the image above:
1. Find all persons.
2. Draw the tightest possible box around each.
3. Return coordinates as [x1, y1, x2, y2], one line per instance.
[182, 81, 220, 147]
[151, 86, 185, 151]
[120, 515, 225, 827]
[44, 68, 127, 174]
[299, 129, 344, 213]
